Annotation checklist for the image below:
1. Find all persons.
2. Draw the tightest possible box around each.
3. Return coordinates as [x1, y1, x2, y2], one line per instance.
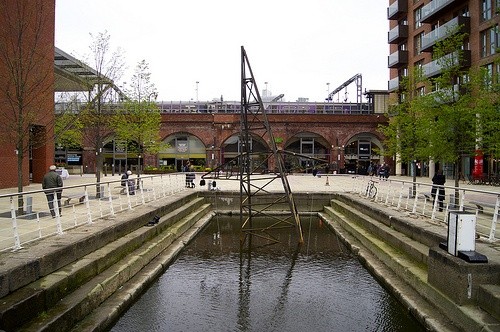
[185, 161, 196, 188]
[120, 170, 134, 195]
[429, 169, 447, 211]
[42, 165, 63, 218]
[368, 160, 391, 181]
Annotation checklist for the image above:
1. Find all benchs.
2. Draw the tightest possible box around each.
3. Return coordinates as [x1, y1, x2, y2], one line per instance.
[61, 193, 86, 208]
[470, 200, 500, 216]
[419, 192, 432, 201]
[114, 186, 127, 195]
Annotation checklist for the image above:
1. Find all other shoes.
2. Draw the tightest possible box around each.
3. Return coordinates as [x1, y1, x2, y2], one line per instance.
[53, 212, 62, 218]
[439, 208, 443, 212]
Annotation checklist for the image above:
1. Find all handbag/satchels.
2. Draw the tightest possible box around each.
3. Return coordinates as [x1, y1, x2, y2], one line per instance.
[52, 191, 61, 199]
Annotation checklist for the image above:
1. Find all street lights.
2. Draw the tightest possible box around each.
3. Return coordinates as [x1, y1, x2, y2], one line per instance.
[195, 81, 200, 101]
[326, 82, 330, 104]
[264, 82, 268, 100]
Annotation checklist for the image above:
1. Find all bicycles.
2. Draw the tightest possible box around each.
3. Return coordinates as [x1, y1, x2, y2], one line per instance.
[366, 179, 379, 198]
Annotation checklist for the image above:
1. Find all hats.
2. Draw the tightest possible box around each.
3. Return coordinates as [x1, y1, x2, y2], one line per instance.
[50, 165, 56, 170]
